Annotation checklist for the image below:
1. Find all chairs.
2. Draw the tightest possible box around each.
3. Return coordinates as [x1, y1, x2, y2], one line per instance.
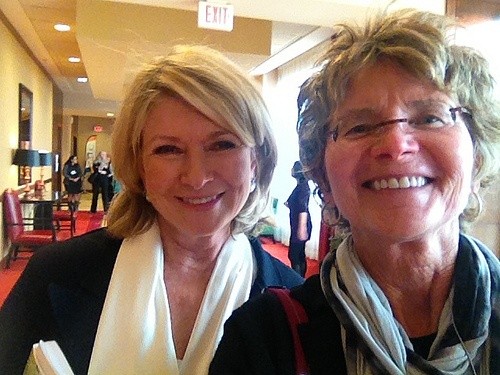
[3, 188, 54, 270]
[35, 180, 78, 238]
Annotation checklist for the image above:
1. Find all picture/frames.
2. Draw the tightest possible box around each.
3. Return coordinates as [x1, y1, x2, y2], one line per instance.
[18, 83, 33, 186]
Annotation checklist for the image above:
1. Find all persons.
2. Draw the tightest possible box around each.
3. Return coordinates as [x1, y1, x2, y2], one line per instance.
[62, 155, 84, 211]
[207, 7, 500, 375]
[90, 151, 114, 213]
[285, 161, 312, 278]
[0, 44, 306, 375]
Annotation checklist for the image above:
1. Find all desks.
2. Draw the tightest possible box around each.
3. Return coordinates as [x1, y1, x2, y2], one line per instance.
[18, 191, 61, 230]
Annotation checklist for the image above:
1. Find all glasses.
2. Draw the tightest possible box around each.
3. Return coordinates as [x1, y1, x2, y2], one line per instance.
[325, 103, 472, 144]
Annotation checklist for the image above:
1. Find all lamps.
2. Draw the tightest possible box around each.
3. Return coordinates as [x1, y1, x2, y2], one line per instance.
[12, 149, 52, 199]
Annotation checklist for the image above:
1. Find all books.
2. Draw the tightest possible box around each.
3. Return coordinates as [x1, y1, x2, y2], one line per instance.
[23, 340, 74, 375]
